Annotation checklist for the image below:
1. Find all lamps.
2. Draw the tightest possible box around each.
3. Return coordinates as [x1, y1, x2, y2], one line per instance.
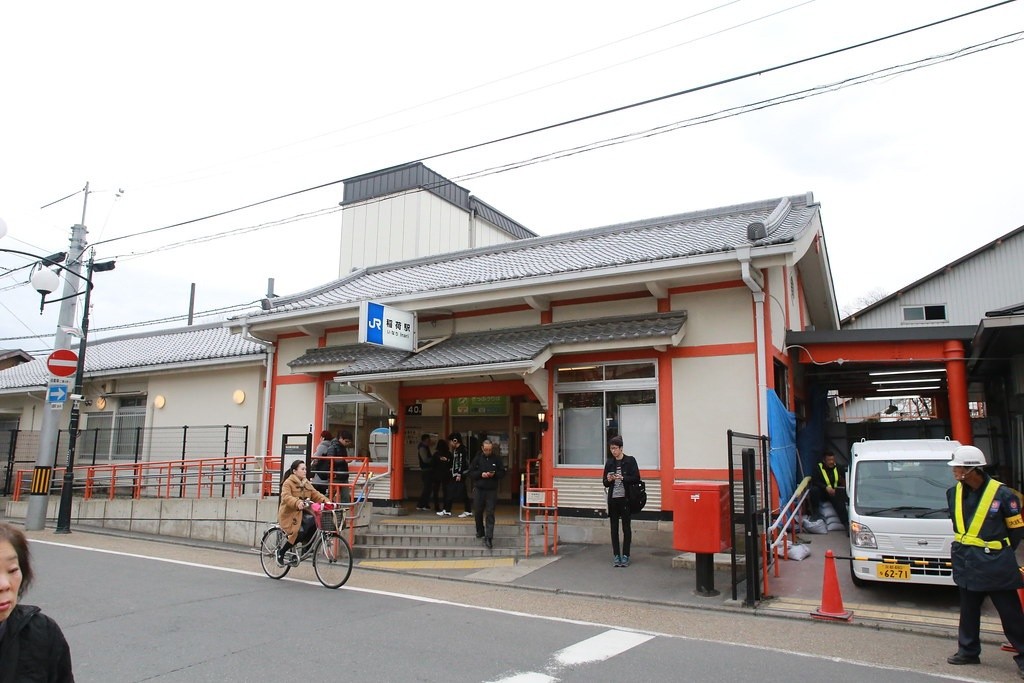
[154, 394, 166, 409]
[96, 398, 106, 410]
[535, 407, 549, 432]
[386, 411, 399, 434]
[232, 389, 245, 404]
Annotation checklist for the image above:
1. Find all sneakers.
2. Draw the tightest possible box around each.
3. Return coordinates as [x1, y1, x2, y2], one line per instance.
[947, 653, 980, 664]
[436, 509, 451, 516]
[275, 550, 285, 567]
[621, 555, 631, 567]
[613, 555, 622, 567]
[458, 511, 473, 517]
[302, 549, 321, 555]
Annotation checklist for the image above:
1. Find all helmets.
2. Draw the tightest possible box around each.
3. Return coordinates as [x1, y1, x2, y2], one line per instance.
[946, 445, 987, 466]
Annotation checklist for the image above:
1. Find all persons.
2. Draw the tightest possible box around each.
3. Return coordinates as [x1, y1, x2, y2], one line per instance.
[310, 430, 333, 495]
[0, 517, 75, 683]
[945, 445, 1024, 678]
[275, 459, 332, 568]
[468, 439, 506, 547]
[416, 433, 433, 510]
[436, 431, 473, 518]
[603, 436, 640, 567]
[322, 431, 352, 507]
[432, 439, 452, 512]
[816, 451, 849, 537]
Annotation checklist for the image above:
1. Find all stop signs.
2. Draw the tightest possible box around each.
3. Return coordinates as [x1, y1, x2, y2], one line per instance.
[46, 349, 80, 378]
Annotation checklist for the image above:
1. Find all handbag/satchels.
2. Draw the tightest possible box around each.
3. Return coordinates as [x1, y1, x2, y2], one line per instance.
[321, 502, 346, 535]
[630, 480, 647, 514]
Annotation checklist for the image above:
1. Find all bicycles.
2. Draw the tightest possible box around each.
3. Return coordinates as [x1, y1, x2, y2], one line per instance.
[253, 499, 357, 589]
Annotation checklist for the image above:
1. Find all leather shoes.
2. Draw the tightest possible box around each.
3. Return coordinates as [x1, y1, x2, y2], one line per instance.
[476, 533, 485, 537]
[485, 536, 492, 547]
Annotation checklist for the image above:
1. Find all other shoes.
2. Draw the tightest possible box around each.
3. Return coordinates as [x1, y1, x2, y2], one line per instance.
[416, 503, 424, 510]
[423, 505, 431, 511]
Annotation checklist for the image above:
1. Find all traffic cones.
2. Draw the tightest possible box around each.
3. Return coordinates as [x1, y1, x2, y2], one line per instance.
[809, 550, 855, 622]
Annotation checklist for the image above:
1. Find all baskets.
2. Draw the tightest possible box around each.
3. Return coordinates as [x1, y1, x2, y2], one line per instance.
[321, 509, 349, 530]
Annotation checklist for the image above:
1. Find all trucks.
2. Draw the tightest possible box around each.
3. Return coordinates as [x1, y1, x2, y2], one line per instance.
[846, 437, 974, 597]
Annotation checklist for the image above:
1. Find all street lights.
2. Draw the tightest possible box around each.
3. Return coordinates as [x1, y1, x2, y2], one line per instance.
[46, 244, 116, 539]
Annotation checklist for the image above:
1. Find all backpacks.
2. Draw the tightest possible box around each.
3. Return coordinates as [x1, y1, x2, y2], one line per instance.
[316, 443, 330, 480]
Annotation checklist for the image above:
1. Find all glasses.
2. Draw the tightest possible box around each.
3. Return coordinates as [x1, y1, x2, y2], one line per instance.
[610, 445, 619, 450]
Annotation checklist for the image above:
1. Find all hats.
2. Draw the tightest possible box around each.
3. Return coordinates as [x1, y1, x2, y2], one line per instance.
[321, 431, 332, 439]
